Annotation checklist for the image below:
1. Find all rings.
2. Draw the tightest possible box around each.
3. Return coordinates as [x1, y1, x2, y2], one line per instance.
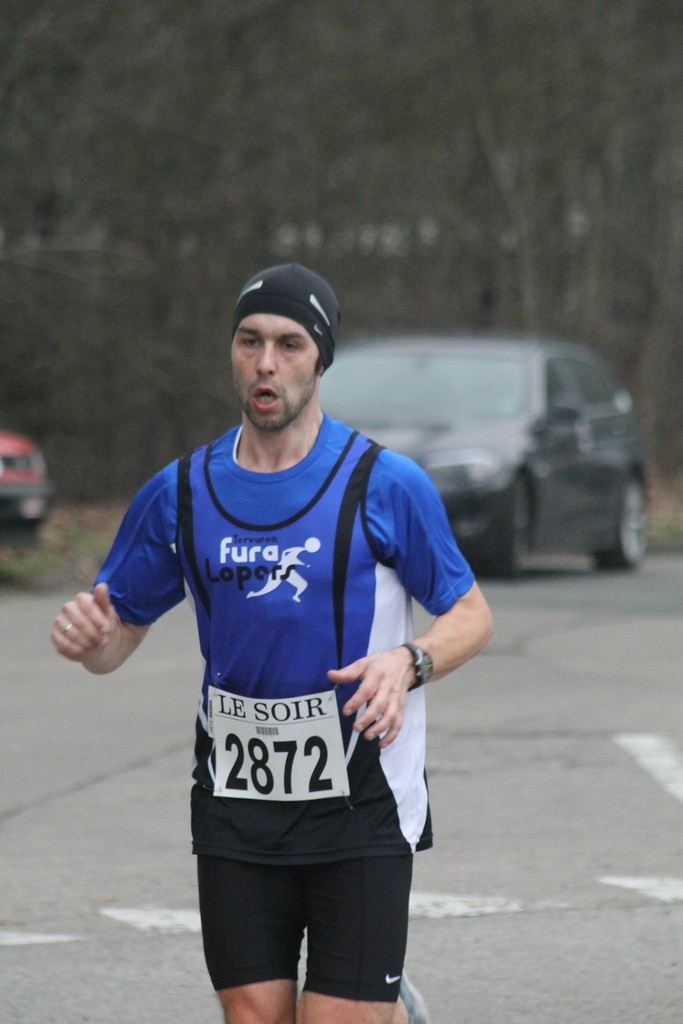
[63, 624, 74, 634]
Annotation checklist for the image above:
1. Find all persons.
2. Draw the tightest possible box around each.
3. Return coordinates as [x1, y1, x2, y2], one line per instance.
[52, 264, 493, 1024]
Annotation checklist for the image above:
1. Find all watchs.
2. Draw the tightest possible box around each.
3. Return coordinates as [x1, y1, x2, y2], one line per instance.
[399, 644, 433, 693]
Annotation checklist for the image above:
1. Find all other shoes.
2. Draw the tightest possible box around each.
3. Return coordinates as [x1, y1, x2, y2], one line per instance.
[395, 973, 429, 1024]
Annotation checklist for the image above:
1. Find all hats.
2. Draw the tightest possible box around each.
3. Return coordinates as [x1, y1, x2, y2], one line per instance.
[230, 262, 337, 372]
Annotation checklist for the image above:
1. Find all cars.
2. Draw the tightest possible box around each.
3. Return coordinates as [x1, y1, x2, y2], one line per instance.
[307, 331, 654, 575]
[0, 426, 56, 534]
